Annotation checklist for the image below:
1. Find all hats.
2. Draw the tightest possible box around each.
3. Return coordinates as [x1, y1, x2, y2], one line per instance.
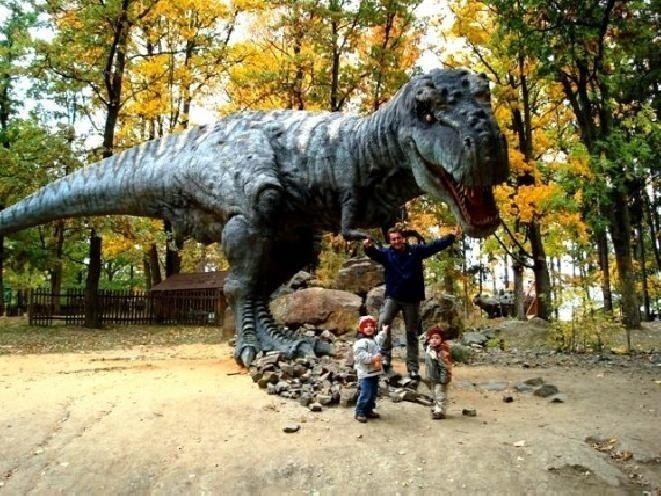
[426, 326, 444, 342]
[359, 316, 377, 333]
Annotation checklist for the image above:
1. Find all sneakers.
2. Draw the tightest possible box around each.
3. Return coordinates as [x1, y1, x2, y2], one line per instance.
[368, 412, 380, 418]
[432, 411, 444, 419]
[409, 370, 420, 379]
[382, 360, 390, 367]
[355, 415, 367, 421]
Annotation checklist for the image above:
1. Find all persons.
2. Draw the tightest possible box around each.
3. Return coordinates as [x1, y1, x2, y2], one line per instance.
[423, 325, 452, 419]
[353, 315, 389, 423]
[364, 227, 459, 381]
